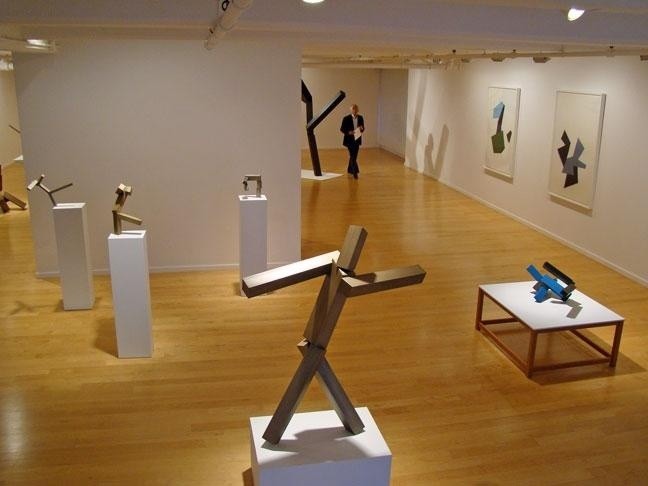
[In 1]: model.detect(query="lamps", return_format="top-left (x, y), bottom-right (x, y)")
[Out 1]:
top-left (201, 0), bottom-right (249, 51)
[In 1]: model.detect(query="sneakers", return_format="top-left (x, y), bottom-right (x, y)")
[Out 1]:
top-left (348, 169), bottom-right (358, 179)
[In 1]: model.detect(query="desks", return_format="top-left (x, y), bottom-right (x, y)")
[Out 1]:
top-left (474, 278), bottom-right (625, 378)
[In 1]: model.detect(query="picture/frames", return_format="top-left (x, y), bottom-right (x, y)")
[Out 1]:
top-left (482, 86), bottom-right (520, 179)
top-left (548, 88), bottom-right (607, 211)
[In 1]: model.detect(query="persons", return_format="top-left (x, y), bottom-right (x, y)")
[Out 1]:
top-left (339, 104), bottom-right (365, 179)
